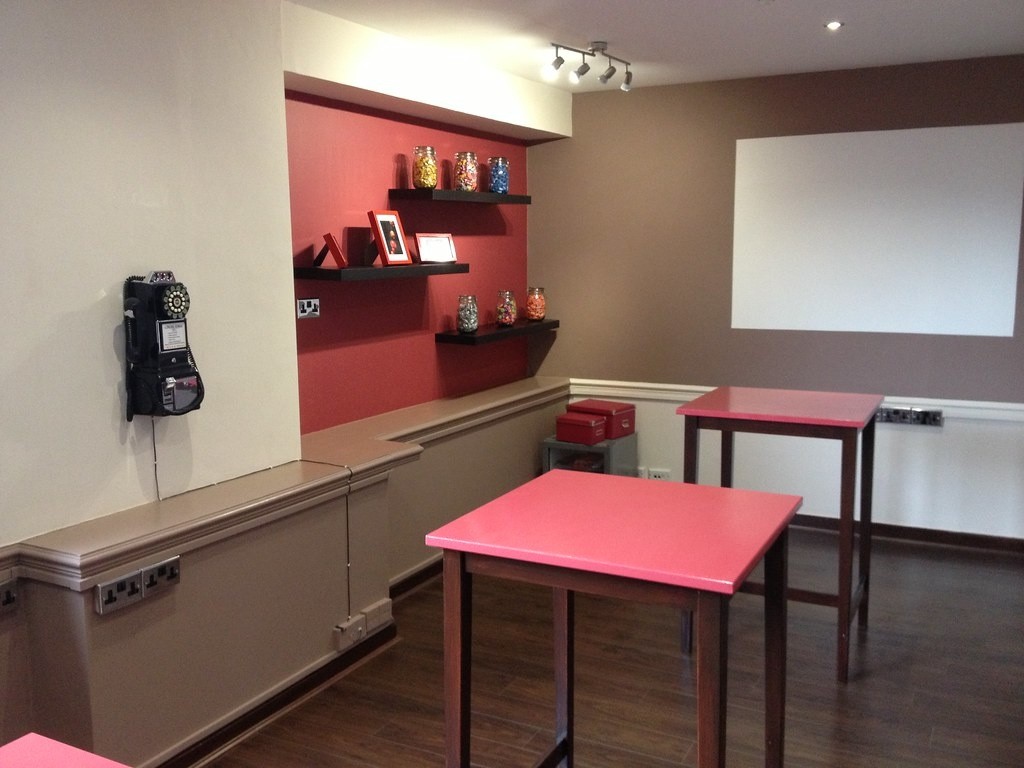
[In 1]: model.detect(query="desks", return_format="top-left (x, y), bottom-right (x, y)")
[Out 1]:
top-left (677, 386), bottom-right (885, 682)
top-left (426, 469), bottom-right (803, 768)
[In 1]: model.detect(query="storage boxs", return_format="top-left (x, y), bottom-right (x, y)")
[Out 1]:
top-left (565, 399), bottom-right (636, 439)
top-left (555, 414), bottom-right (606, 446)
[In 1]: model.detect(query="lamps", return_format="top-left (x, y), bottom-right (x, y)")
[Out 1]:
top-left (544, 41), bottom-right (632, 91)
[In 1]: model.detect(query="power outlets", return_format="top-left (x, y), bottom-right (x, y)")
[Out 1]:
top-left (649, 469), bottom-right (672, 482)
top-left (622, 467), bottom-right (648, 480)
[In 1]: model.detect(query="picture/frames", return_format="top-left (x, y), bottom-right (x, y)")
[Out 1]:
top-left (414, 233), bottom-right (458, 263)
top-left (314, 233), bottom-right (349, 268)
top-left (365, 210), bottom-right (413, 266)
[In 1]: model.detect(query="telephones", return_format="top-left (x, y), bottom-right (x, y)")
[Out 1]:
top-left (124, 270), bottom-right (206, 422)
